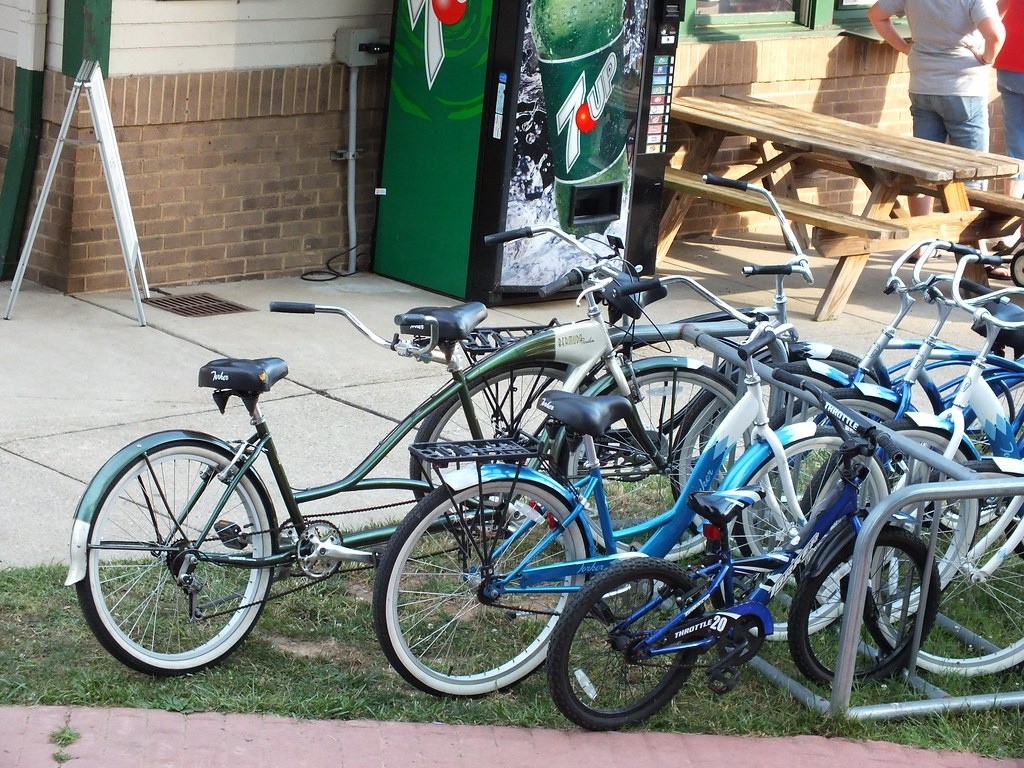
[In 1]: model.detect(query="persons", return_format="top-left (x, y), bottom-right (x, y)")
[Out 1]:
top-left (867, 0), bottom-right (1011, 282)
top-left (995, 0), bottom-right (1024, 160)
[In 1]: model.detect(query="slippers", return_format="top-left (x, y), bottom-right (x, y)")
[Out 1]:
top-left (984, 264), bottom-right (1014, 280)
top-left (908, 247), bottom-right (941, 265)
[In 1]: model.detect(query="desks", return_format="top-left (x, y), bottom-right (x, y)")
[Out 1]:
top-left (667, 89), bottom-right (1023, 324)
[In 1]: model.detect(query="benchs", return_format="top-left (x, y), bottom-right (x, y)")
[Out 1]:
top-left (748, 139), bottom-right (1024, 253)
top-left (655, 166), bottom-right (910, 324)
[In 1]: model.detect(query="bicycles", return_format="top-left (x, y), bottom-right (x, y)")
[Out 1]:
top-left (61, 173), bottom-right (1024, 731)
